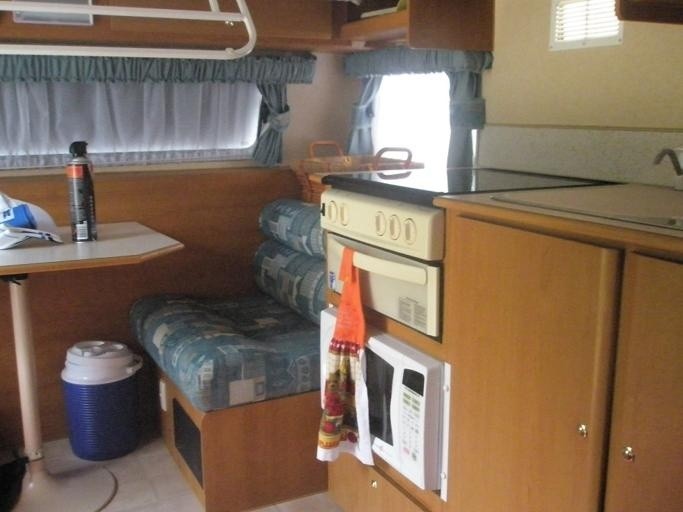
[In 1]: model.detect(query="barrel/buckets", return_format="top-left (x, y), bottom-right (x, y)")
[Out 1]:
top-left (61, 340), bottom-right (144, 461)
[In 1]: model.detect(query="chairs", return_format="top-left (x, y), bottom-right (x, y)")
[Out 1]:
top-left (125, 198), bottom-right (327, 511)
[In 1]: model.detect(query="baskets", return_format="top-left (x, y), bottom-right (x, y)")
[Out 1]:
top-left (290, 155), bottom-right (424, 205)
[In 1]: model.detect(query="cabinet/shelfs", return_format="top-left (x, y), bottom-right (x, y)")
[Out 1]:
top-left (0, 0), bottom-right (495, 53)
top-left (431, 186), bottom-right (683, 512)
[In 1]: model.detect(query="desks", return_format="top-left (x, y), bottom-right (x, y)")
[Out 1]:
top-left (0, 219), bottom-right (183, 512)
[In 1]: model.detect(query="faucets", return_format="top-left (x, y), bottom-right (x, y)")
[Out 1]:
top-left (653, 150), bottom-right (683, 176)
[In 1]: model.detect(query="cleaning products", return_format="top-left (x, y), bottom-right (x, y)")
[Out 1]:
top-left (66, 141), bottom-right (98, 242)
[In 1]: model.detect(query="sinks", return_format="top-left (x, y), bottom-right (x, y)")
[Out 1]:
top-left (490, 181), bottom-right (683, 235)
